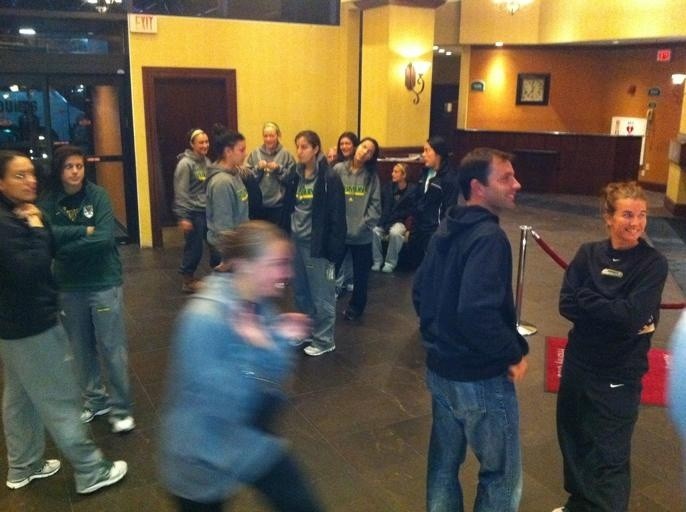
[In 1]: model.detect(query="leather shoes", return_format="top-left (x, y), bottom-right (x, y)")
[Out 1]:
top-left (343, 306), bottom-right (358, 321)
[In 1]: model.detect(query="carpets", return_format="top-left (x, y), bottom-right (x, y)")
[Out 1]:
top-left (544, 335), bottom-right (672, 407)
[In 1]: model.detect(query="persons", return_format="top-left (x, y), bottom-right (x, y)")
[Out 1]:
top-left (0, 148), bottom-right (129, 494)
top-left (149, 222), bottom-right (330, 512)
top-left (172, 128), bottom-right (212, 292)
top-left (34, 146), bottom-right (137, 435)
top-left (413, 135), bottom-right (460, 276)
top-left (281, 130), bottom-right (380, 358)
top-left (71, 115), bottom-right (97, 171)
top-left (550, 182), bottom-right (669, 512)
top-left (408, 145), bottom-right (529, 512)
top-left (371, 163), bottom-right (417, 271)
top-left (204, 121), bottom-right (296, 266)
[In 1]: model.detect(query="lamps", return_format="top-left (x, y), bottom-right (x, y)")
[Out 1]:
top-left (405, 61), bottom-right (429, 104)
top-left (490, 0), bottom-right (538, 15)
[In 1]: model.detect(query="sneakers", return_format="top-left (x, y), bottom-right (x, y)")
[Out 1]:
top-left (182, 272), bottom-right (195, 292)
top-left (302, 342), bottom-right (336, 357)
top-left (81, 403), bottom-right (112, 423)
top-left (287, 333), bottom-right (312, 347)
top-left (371, 261), bottom-right (383, 272)
top-left (76, 460), bottom-right (128, 494)
top-left (108, 414), bottom-right (135, 434)
top-left (381, 264), bottom-right (396, 273)
top-left (5, 458), bottom-right (61, 490)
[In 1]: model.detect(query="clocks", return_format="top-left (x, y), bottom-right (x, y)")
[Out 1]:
top-left (515, 73), bottom-right (551, 106)
